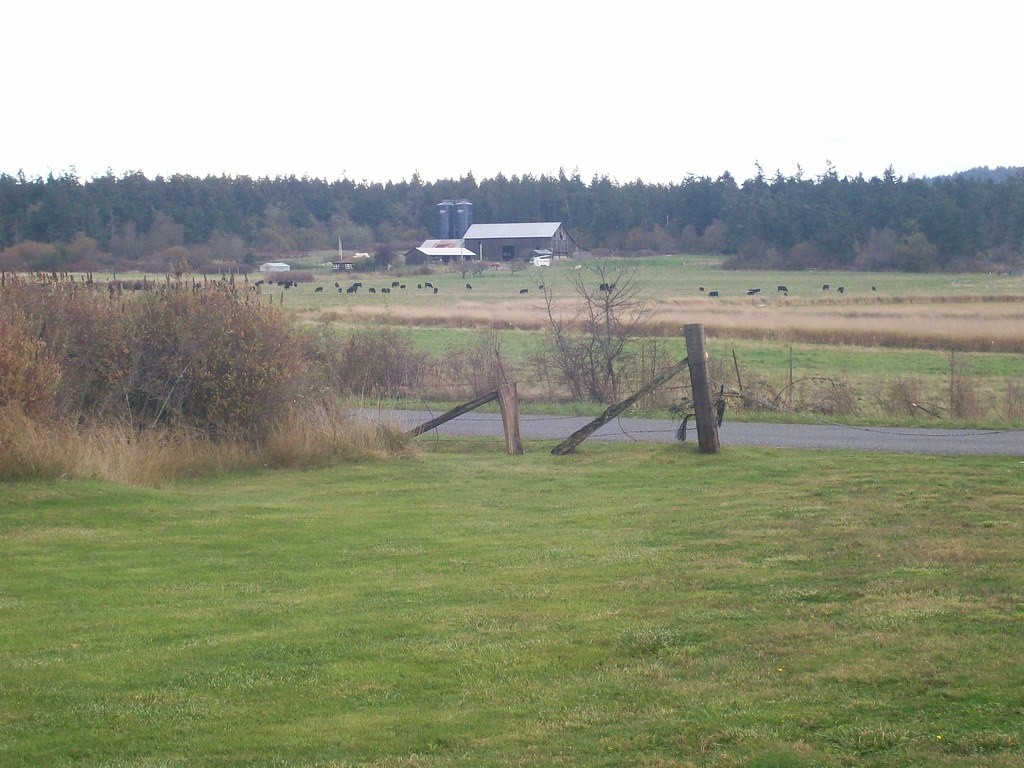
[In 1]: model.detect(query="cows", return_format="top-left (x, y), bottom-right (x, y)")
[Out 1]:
top-left (872, 286), bottom-right (876, 291)
top-left (699, 286), bottom-right (719, 298)
top-left (539, 284), bottom-right (544, 289)
top-left (519, 288), bottom-right (528, 294)
top-left (778, 285), bottom-right (788, 297)
top-left (465, 283), bottom-right (473, 289)
top-left (746, 288), bottom-right (761, 296)
top-left (315, 282), bottom-right (438, 294)
top-left (822, 283), bottom-right (845, 294)
top-left (599, 283), bottom-right (616, 291)
top-left (254, 280), bottom-right (297, 290)
